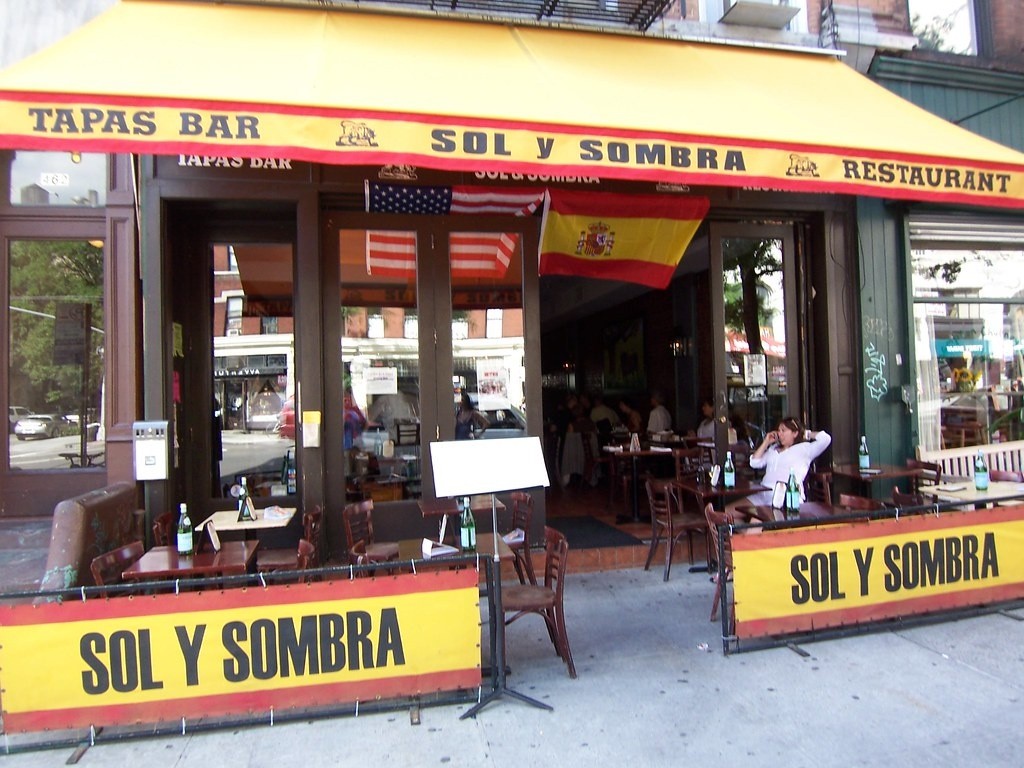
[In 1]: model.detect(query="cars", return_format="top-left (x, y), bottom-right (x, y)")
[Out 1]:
top-left (936, 384), bottom-right (1024, 438)
top-left (8, 406), bottom-right (98, 440)
top-left (275, 390), bottom-right (527, 440)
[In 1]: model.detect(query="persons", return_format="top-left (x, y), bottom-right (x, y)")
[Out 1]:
top-left (344, 387), bottom-right (368, 450)
top-left (709, 416), bottom-right (832, 583)
top-left (455, 393), bottom-right (490, 439)
top-left (540, 394), bottom-right (715, 441)
top-left (937, 358), bottom-right (956, 392)
top-left (362, 391), bottom-right (415, 443)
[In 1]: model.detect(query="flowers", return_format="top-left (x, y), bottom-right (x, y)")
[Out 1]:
top-left (951, 368), bottom-right (983, 392)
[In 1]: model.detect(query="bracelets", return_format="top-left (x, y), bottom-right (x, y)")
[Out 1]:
top-left (805, 430), bottom-right (811, 440)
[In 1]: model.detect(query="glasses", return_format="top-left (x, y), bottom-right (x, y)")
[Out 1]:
top-left (787, 417), bottom-right (799, 429)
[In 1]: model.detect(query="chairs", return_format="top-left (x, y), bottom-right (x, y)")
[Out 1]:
top-left (90, 421), bottom-right (1024, 677)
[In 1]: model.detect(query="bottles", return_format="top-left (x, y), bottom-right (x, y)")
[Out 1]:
top-left (374, 428), bottom-right (383, 457)
top-left (281, 455), bottom-right (288, 485)
top-left (786, 466), bottom-right (800, 512)
top-left (974, 448), bottom-right (988, 490)
top-left (460, 496), bottom-right (477, 551)
top-left (724, 451), bottom-right (735, 489)
top-left (406, 451), bottom-right (416, 481)
top-left (176, 503), bottom-right (194, 556)
top-left (238, 476), bottom-right (250, 517)
top-left (858, 435), bottom-right (870, 469)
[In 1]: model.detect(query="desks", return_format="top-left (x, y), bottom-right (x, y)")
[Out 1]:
top-left (395, 419), bottom-right (419, 446)
top-left (194, 507), bottom-right (297, 586)
top-left (417, 493), bottom-right (509, 534)
top-left (121, 538), bottom-right (259, 594)
top-left (603, 441), bottom-right (673, 523)
top-left (374, 471), bottom-right (420, 503)
top-left (397, 532), bottom-right (517, 567)
top-left (670, 435), bottom-right (713, 448)
top-left (833, 463), bottom-right (923, 497)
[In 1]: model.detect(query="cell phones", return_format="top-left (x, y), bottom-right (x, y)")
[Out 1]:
top-left (774, 434), bottom-right (779, 441)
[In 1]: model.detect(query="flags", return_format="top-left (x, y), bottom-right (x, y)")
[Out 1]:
top-left (537, 188), bottom-right (710, 290)
top-left (366, 231), bottom-right (519, 279)
top-left (364, 179), bottom-right (546, 217)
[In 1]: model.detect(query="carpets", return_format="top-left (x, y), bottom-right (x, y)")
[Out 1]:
top-left (544, 514), bottom-right (642, 549)
